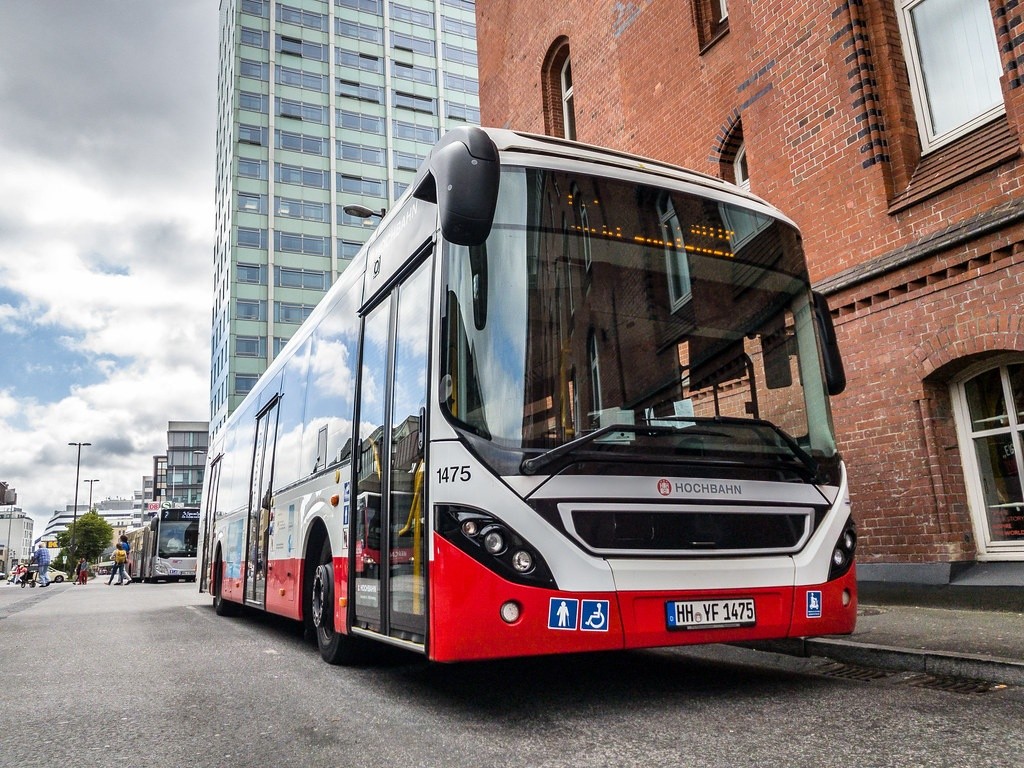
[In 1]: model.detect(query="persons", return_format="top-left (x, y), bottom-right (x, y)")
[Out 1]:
top-left (30, 544), bottom-right (50, 587)
top-left (11, 565), bottom-right (27, 583)
top-left (167, 533), bottom-right (183, 552)
top-left (114, 535), bottom-right (132, 585)
top-left (73, 557), bottom-right (88, 585)
top-left (104, 543), bottom-right (128, 585)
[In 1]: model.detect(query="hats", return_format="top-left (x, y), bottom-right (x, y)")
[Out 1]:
top-left (20, 565), bottom-right (24, 568)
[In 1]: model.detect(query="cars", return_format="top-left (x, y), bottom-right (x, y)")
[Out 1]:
top-left (7, 562), bottom-right (68, 583)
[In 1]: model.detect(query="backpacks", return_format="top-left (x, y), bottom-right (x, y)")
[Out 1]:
top-left (115, 549), bottom-right (126, 563)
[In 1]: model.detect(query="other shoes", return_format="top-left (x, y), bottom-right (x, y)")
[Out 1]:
top-left (114, 583), bottom-right (121, 585)
top-left (104, 582), bottom-right (110, 585)
top-left (39, 585), bottom-right (46, 587)
top-left (124, 580), bottom-right (133, 585)
top-left (73, 582), bottom-right (86, 585)
top-left (46, 582), bottom-right (50, 586)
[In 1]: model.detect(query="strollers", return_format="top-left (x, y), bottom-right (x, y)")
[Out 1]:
top-left (20, 563), bottom-right (39, 588)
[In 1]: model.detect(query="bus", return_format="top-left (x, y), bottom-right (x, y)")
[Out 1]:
top-left (194, 124), bottom-right (858, 664)
top-left (118, 508), bottom-right (201, 583)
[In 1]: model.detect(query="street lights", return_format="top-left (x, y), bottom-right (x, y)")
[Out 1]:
top-left (68, 442), bottom-right (91, 580)
top-left (84, 479), bottom-right (100, 561)
